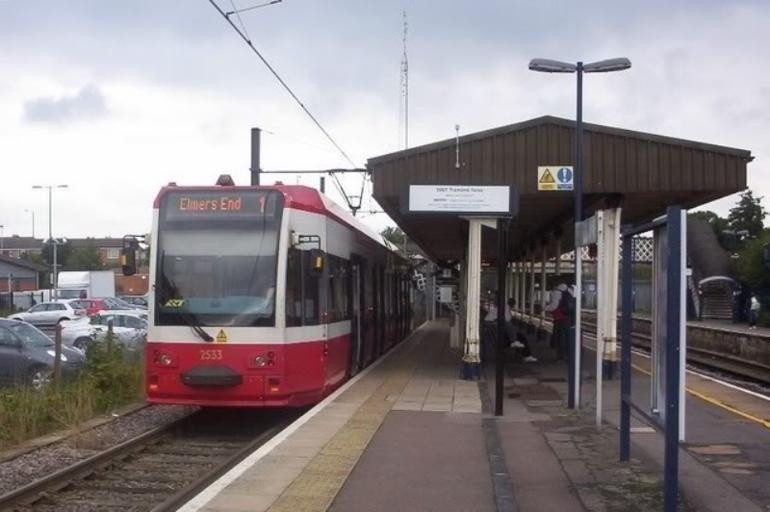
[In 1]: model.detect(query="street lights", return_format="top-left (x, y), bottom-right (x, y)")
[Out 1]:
top-left (31, 183), bottom-right (69, 286)
top-left (529, 57), bottom-right (633, 414)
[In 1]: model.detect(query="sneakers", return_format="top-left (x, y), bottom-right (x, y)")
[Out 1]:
top-left (510, 341), bottom-right (525, 348)
top-left (522, 356), bottom-right (537, 362)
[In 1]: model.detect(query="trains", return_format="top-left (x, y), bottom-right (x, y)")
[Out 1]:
top-left (120, 128), bottom-right (416, 410)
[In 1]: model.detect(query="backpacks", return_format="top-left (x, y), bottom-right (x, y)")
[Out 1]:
top-left (553, 287), bottom-right (575, 313)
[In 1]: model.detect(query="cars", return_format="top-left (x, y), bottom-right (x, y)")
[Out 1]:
top-left (0, 268), bottom-right (149, 395)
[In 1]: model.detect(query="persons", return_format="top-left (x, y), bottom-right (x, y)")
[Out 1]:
top-left (484, 298), bottom-right (515, 330)
top-left (544, 276), bottom-right (576, 363)
top-left (744, 289), bottom-right (762, 331)
top-left (504, 327), bottom-right (540, 363)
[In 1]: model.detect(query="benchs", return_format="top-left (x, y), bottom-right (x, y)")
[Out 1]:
top-left (489, 330), bottom-right (522, 364)
